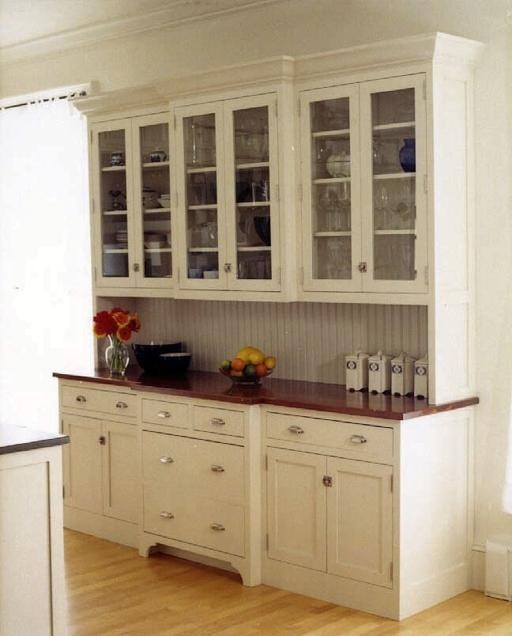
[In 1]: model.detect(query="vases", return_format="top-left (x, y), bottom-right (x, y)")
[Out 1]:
top-left (104, 335), bottom-right (130, 377)
top-left (399, 137), bottom-right (416, 173)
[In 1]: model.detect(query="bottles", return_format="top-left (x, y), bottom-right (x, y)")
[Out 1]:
top-left (184, 123), bottom-right (213, 165)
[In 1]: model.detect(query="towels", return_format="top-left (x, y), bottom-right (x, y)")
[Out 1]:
top-left (483, 540), bottom-right (511, 601)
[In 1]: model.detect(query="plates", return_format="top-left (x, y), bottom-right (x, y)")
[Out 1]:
top-left (115, 228), bottom-right (128, 249)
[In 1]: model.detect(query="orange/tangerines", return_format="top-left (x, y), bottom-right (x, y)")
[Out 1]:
top-left (221, 359), bottom-right (231, 371)
top-left (245, 364), bottom-right (255, 376)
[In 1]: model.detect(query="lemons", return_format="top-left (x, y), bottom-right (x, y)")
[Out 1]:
top-left (264, 356), bottom-right (276, 370)
top-left (249, 352), bottom-right (265, 364)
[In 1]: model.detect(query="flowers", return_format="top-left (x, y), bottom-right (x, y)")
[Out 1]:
top-left (92, 304), bottom-right (141, 342)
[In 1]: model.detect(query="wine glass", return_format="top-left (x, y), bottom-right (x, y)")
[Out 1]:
top-left (109, 183), bottom-right (122, 210)
top-left (316, 182), bottom-right (351, 232)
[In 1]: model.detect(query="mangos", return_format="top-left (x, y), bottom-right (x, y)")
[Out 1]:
top-left (237, 347), bottom-right (260, 363)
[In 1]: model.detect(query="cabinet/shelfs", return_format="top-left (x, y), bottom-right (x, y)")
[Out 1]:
top-left (69, 77), bottom-right (175, 300)
top-left (174, 55), bottom-right (290, 303)
top-left (259, 396), bottom-right (480, 623)
top-left (52, 372), bottom-right (141, 556)
top-left (132, 382), bottom-right (263, 588)
top-left (0, 426), bottom-right (72, 636)
top-left (294, 30), bottom-right (485, 306)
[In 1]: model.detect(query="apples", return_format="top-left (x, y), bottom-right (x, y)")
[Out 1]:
top-left (231, 358), bottom-right (245, 371)
top-left (256, 364), bottom-right (267, 376)
top-left (230, 370), bottom-right (243, 377)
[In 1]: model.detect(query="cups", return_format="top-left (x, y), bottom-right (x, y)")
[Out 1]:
top-left (112, 151), bottom-right (123, 165)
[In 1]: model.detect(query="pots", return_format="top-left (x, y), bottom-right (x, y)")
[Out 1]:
top-left (144, 186), bottom-right (158, 208)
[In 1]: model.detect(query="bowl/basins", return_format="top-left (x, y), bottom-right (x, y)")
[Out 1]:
top-left (203, 271), bottom-right (218, 279)
top-left (157, 194), bottom-right (170, 207)
top-left (189, 269), bottom-right (202, 277)
top-left (251, 216), bottom-right (270, 246)
top-left (143, 231), bottom-right (166, 249)
top-left (132, 341), bottom-right (180, 373)
top-left (161, 353), bottom-right (192, 371)
